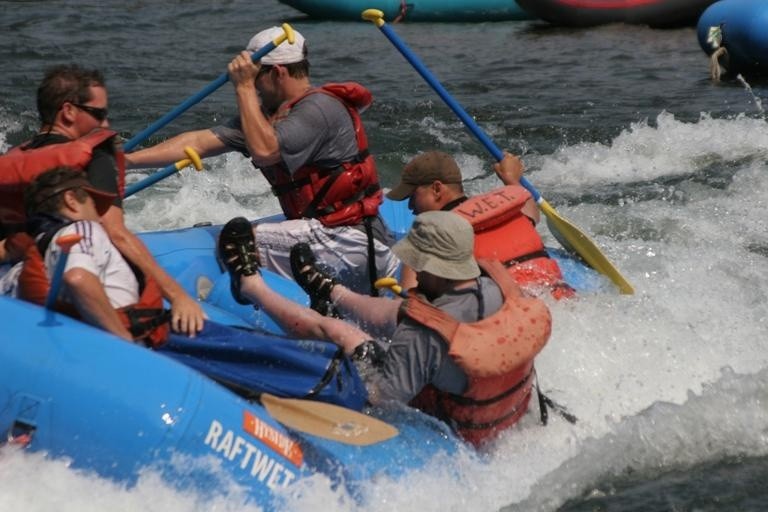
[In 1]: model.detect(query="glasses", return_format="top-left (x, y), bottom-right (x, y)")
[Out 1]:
top-left (77, 103), bottom-right (108, 122)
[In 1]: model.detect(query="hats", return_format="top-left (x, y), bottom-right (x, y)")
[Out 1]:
top-left (27, 165), bottom-right (118, 218)
top-left (386, 153), bottom-right (462, 202)
top-left (247, 25), bottom-right (306, 66)
top-left (391, 209), bottom-right (482, 281)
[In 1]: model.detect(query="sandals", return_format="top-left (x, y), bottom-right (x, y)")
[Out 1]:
top-left (290, 242), bottom-right (340, 319)
top-left (217, 216), bottom-right (262, 304)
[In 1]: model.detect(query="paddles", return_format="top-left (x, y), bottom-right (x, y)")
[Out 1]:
top-left (213, 369), bottom-right (400, 447)
top-left (362, 8), bottom-right (634, 297)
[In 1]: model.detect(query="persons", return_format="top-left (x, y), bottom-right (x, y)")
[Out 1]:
top-left (120, 21), bottom-right (405, 293)
top-left (22, 163), bottom-right (171, 346)
top-left (2, 57), bottom-right (211, 341)
top-left (214, 206), bottom-right (552, 450)
top-left (368, 142), bottom-right (583, 303)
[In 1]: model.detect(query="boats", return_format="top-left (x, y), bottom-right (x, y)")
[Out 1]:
top-left (514, 0), bottom-right (707, 24)
top-left (697, 0), bottom-right (768, 73)
top-left (0, 192), bottom-right (613, 511)
top-left (277, 0), bottom-right (528, 22)
